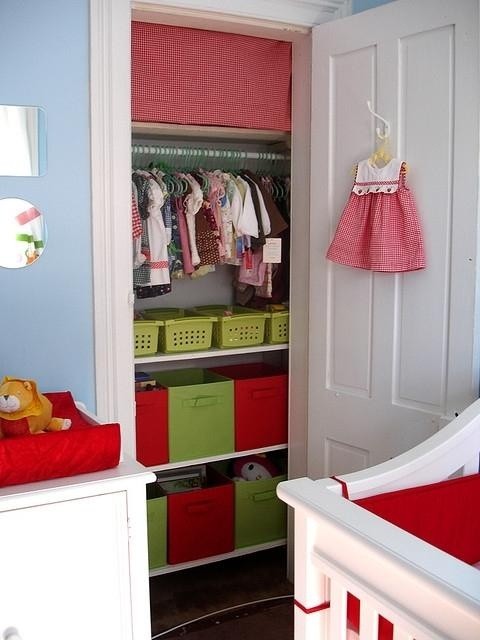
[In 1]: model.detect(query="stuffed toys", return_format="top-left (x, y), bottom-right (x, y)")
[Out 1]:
top-left (232, 452), bottom-right (281, 483)
top-left (0, 376), bottom-right (72, 434)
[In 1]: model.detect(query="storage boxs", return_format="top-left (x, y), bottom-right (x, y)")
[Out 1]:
top-left (160, 466), bottom-right (232, 566)
top-left (208, 358), bottom-right (289, 452)
top-left (152, 366), bottom-right (235, 464)
top-left (135, 383), bottom-right (169, 467)
top-left (228, 458), bottom-right (287, 549)
top-left (145, 482), bottom-right (168, 570)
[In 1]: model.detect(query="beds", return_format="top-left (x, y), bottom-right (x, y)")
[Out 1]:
top-left (276, 389), bottom-right (480, 640)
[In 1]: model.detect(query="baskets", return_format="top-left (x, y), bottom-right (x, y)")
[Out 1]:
top-left (128, 302), bottom-right (291, 354)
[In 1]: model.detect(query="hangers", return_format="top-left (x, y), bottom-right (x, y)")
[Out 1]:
top-left (353, 132), bottom-right (409, 178)
top-left (132, 142), bottom-right (293, 201)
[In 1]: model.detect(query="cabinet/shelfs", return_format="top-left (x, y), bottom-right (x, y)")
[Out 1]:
top-left (131, 22), bottom-right (289, 639)
top-left (1, 454), bottom-right (157, 640)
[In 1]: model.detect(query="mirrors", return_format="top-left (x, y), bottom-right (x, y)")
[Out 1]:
top-left (1, 106), bottom-right (47, 177)
top-left (0, 197), bottom-right (50, 271)
